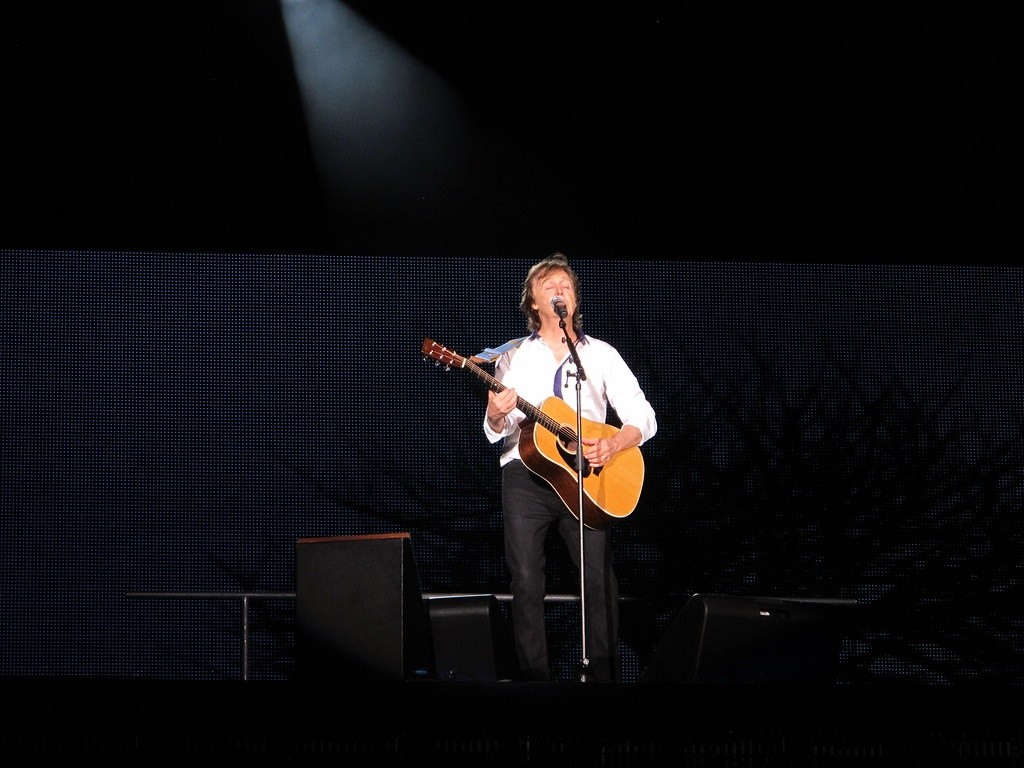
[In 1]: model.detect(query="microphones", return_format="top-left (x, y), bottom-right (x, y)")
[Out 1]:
top-left (551, 296), bottom-right (568, 318)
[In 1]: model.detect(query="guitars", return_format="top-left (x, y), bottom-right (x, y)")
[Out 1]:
top-left (419, 335), bottom-right (646, 534)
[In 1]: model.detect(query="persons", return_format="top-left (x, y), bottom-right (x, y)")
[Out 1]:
top-left (483, 253), bottom-right (658, 683)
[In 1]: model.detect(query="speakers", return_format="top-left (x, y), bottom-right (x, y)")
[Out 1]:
top-left (427, 596), bottom-right (524, 682)
top-left (633, 593), bottom-right (858, 690)
top-left (297, 539), bottom-right (436, 680)
